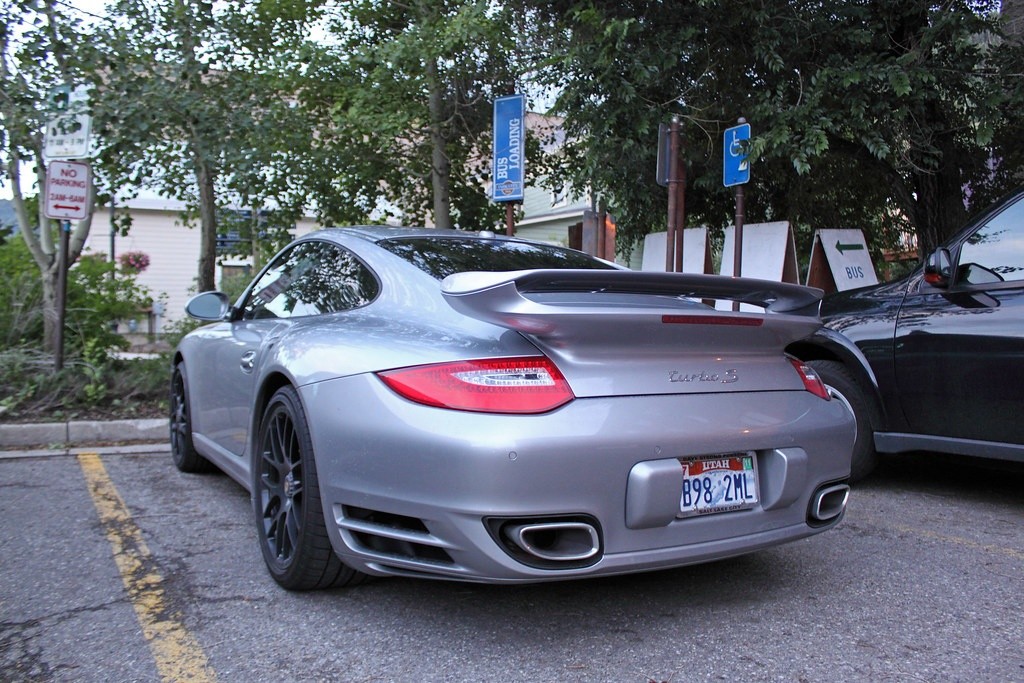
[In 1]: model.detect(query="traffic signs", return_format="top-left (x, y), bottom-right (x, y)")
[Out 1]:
top-left (44, 160), bottom-right (92, 221)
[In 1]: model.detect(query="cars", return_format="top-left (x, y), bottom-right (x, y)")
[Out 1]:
top-left (786, 183), bottom-right (1024, 485)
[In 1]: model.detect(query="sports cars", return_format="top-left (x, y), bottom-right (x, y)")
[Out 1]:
top-left (168, 223), bottom-right (860, 593)
